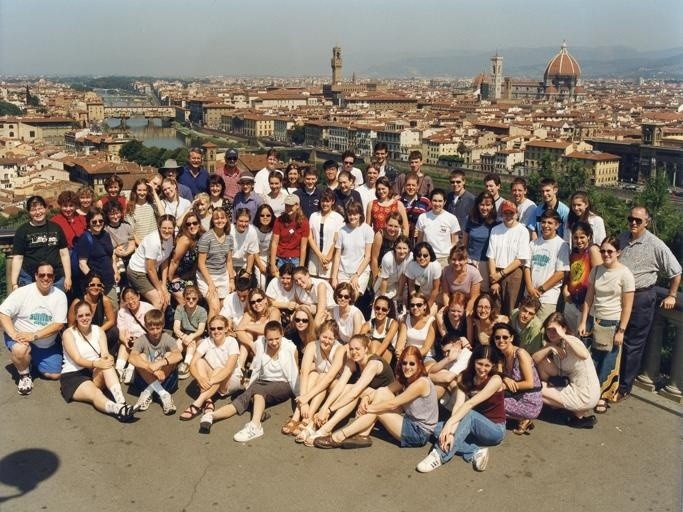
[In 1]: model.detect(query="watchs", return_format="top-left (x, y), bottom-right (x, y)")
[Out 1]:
top-left (618, 326), bottom-right (628, 333)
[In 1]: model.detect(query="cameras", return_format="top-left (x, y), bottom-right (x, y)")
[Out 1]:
top-left (129, 336), bottom-right (139, 348)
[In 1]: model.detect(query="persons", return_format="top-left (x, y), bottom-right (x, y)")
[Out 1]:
top-left (295, 333), bottom-right (397, 445)
top-left (1, 141), bottom-right (609, 392)
top-left (609, 203), bottom-right (682, 404)
top-left (489, 322), bottom-right (543, 435)
top-left (313, 345), bottom-right (439, 449)
top-left (578, 234), bottom-right (636, 414)
top-left (416, 344), bottom-right (506, 473)
top-left (128, 308), bottom-right (183, 415)
top-left (531, 311), bottom-right (599, 429)
top-left (179, 314), bottom-right (245, 421)
top-left (281, 318), bottom-right (348, 436)
top-left (60, 301), bottom-right (135, 422)
top-left (199, 320), bottom-right (300, 443)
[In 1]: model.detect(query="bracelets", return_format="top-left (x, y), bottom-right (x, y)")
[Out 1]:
top-left (666, 293), bottom-right (680, 300)
top-left (365, 394), bottom-right (372, 404)
top-left (447, 433), bottom-right (456, 437)
top-left (329, 408), bottom-right (334, 414)
